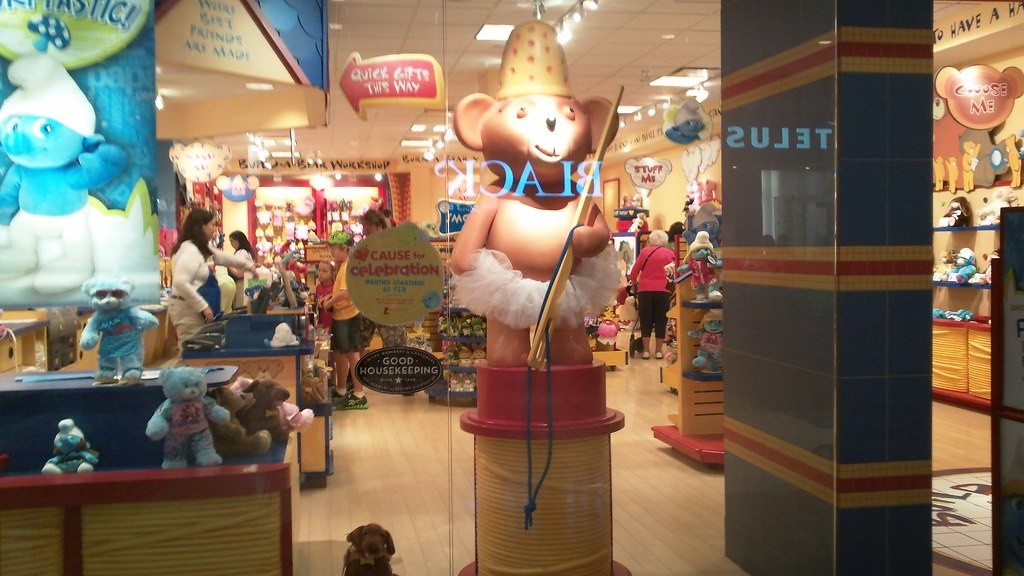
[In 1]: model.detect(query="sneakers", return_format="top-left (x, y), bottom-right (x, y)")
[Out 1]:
top-left (332, 393), bottom-right (347, 406)
top-left (336, 394), bottom-right (368, 410)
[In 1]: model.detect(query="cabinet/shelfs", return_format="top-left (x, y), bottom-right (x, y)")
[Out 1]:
top-left (176, 180), bottom-right (223, 248)
top-left (424, 308), bottom-right (487, 402)
top-left (651, 234), bottom-right (725, 469)
top-left (933, 226), bottom-right (1000, 410)
top-left (179, 309), bottom-right (335, 478)
top-left (0, 304), bottom-right (177, 372)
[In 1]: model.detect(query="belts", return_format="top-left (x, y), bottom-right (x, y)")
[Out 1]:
top-left (172, 295), bottom-right (184, 301)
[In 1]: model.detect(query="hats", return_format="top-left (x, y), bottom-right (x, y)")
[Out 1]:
top-left (322, 231), bottom-right (354, 246)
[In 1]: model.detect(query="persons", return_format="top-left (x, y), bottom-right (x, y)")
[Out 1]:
top-left (169, 206), bottom-right (260, 356)
top-left (317, 230), bottom-right (369, 410)
top-left (362, 210), bottom-right (416, 396)
top-left (315, 258), bottom-right (355, 395)
top-left (630, 229), bottom-right (675, 359)
top-left (228, 231), bottom-right (257, 310)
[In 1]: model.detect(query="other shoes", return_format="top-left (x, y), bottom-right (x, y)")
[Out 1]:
top-left (642, 351), bottom-right (650, 359)
top-left (655, 352), bottom-right (663, 359)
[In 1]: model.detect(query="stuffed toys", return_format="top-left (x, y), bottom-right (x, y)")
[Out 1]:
top-left (41, 420), bottom-right (98, 474)
top-left (932, 184), bottom-right (1017, 325)
top-left (253, 196), bottom-right (384, 307)
top-left (342, 523), bottom-right (400, 576)
top-left (673, 200), bottom-right (724, 373)
top-left (414, 263), bottom-right (487, 391)
top-left (145, 366), bottom-right (231, 469)
top-left (208, 370), bottom-right (313, 459)
top-left (81, 275), bottom-right (158, 383)
